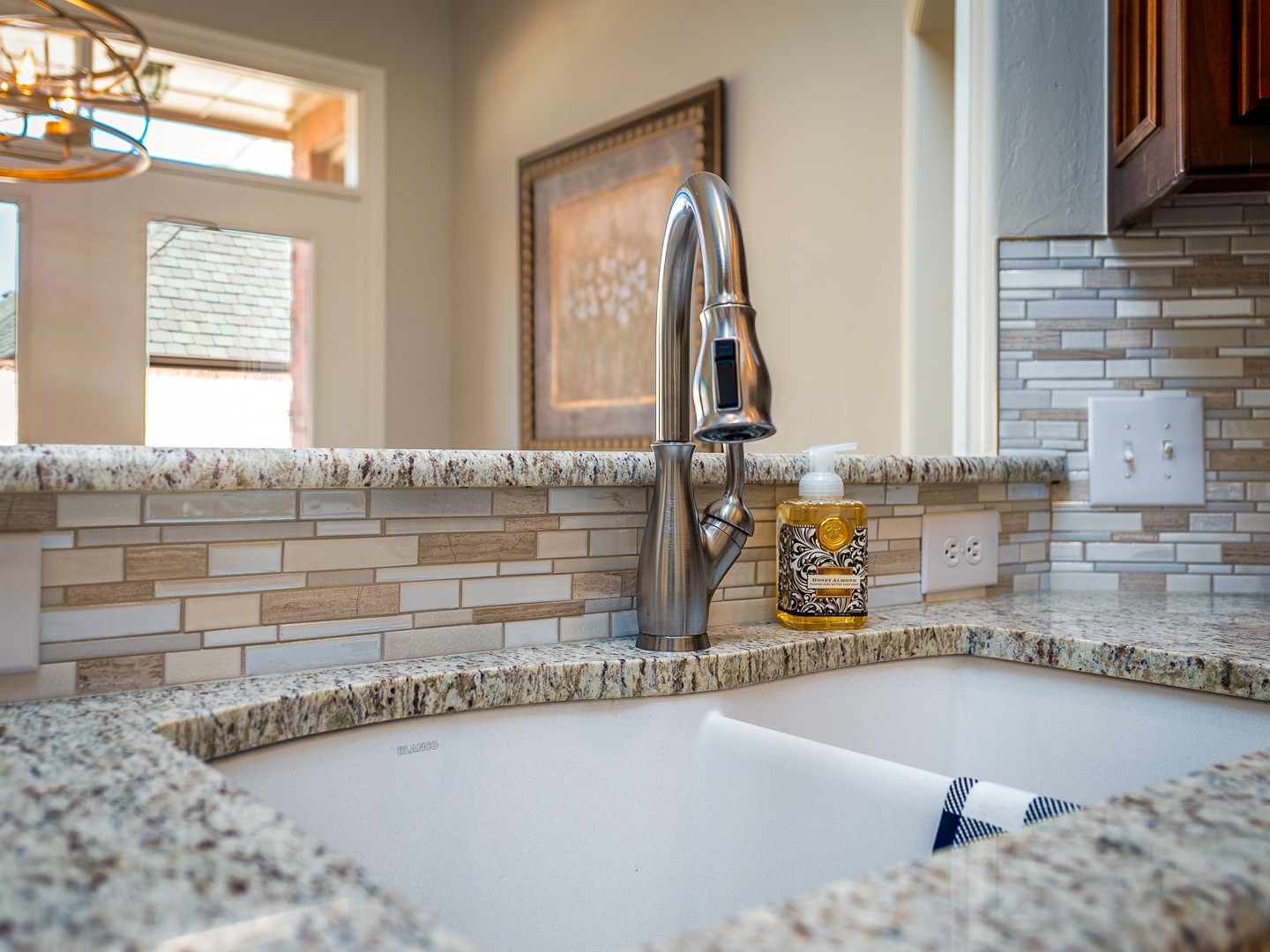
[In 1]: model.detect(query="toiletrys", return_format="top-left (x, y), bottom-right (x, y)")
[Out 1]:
top-left (771, 442), bottom-right (872, 632)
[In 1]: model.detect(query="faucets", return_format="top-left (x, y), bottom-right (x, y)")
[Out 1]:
top-left (632, 169), bottom-right (779, 655)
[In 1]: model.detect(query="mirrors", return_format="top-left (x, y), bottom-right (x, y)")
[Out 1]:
top-left (0, 0), bottom-right (999, 458)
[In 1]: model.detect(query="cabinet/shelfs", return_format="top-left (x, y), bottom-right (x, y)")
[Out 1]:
top-left (1110, 0), bottom-right (1270, 231)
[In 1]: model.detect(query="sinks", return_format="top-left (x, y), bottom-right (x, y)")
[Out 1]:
top-left (145, 658), bottom-right (1097, 952)
top-left (689, 615), bottom-right (1270, 848)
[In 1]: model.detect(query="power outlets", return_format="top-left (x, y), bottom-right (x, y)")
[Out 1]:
top-left (922, 510), bottom-right (999, 594)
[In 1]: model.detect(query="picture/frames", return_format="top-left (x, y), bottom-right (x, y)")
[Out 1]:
top-left (516, 79), bottom-right (724, 459)
